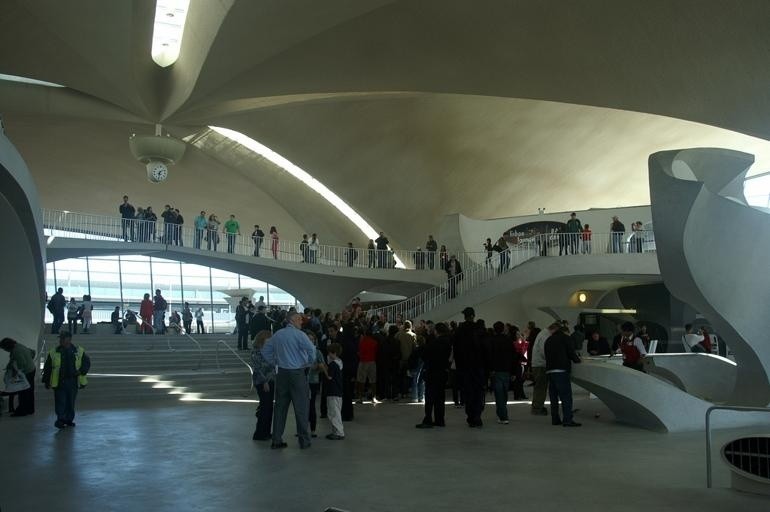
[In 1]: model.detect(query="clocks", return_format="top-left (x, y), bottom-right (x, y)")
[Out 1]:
top-left (144, 162), bottom-right (168, 183)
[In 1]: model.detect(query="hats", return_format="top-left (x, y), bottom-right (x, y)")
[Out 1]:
top-left (461, 307), bottom-right (475, 314)
top-left (57, 330), bottom-right (72, 337)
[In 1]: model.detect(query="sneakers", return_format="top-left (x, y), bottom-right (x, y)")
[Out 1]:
top-left (498, 420), bottom-right (509, 424)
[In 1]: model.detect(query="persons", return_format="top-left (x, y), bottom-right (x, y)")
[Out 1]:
top-left (194, 211), bottom-right (206, 249)
top-left (440, 245), bottom-right (447, 270)
top-left (507, 318), bottom-right (584, 427)
top-left (497, 232), bottom-right (511, 276)
top-left (538, 235), bottom-right (549, 256)
top-left (0, 337), bottom-right (36, 417)
top-left (43, 330), bottom-right (90, 429)
top-left (195, 307), bottom-right (205, 334)
top-left (631, 221), bottom-right (645, 253)
top-left (235, 295), bottom-right (364, 440)
top-left (556, 212), bottom-right (592, 256)
top-left (368, 232), bottom-right (390, 268)
top-left (416, 306), bottom-right (516, 430)
top-left (356, 304), bottom-right (426, 404)
top-left (485, 238), bottom-right (493, 270)
top-left (344, 242), bottom-right (356, 267)
top-left (300, 234), bottom-right (320, 264)
top-left (612, 216), bottom-right (625, 253)
top-left (445, 255), bottom-right (464, 299)
top-left (205, 214), bottom-right (221, 251)
top-left (587, 321), bottom-right (652, 372)
top-left (119, 196), bottom-right (135, 242)
top-left (260, 311), bottom-right (317, 449)
top-left (413, 246), bottom-right (425, 270)
top-left (140, 288), bottom-right (193, 334)
top-left (51, 287), bottom-right (66, 334)
top-left (426, 235), bottom-right (437, 269)
top-left (160, 204), bottom-right (183, 247)
top-left (681, 322), bottom-right (712, 353)
top-left (67, 295), bottom-right (93, 334)
top-left (270, 227), bottom-right (279, 259)
top-left (111, 306), bottom-right (127, 334)
top-left (251, 225), bottom-right (265, 256)
top-left (134, 207), bottom-right (158, 242)
top-left (223, 214), bottom-right (240, 253)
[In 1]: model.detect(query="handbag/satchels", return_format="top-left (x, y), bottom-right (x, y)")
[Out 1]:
top-left (5, 363), bottom-right (31, 393)
top-left (691, 344), bottom-right (706, 352)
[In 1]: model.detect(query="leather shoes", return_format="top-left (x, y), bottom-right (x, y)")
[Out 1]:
top-left (563, 422), bottom-right (581, 426)
top-left (328, 435), bottom-right (344, 440)
top-left (55, 420), bottom-right (65, 428)
top-left (272, 442), bottom-right (287, 449)
top-left (326, 434), bottom-right (334, 438)
top-left (416, 423), bottom-right (432, 428)
top-left (64, 420), bottom-right (75, 426)
